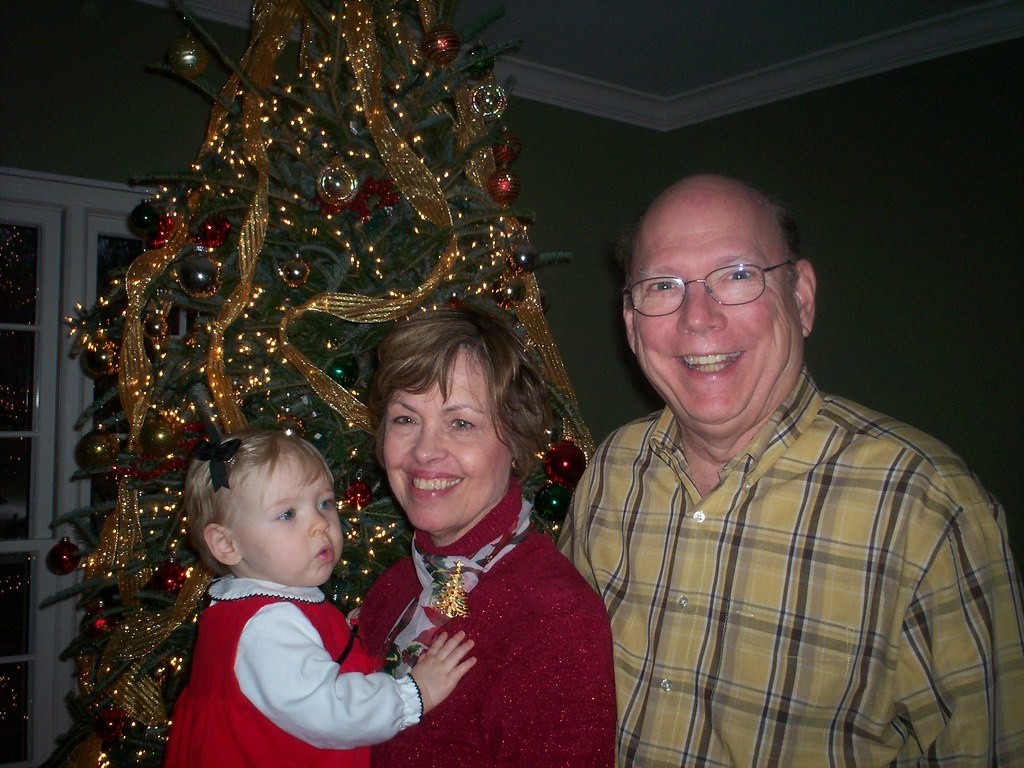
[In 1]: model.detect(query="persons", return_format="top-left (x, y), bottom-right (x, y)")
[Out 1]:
top-left (556, 174), bottom-right (1024, 768)
top-left (165, 432), bottom-right (478, 768)
top-left (355, 305), bottom-right (616, 768)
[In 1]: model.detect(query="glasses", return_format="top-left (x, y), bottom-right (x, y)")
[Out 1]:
top-left (622, 261), bottom-right (792, 317)
